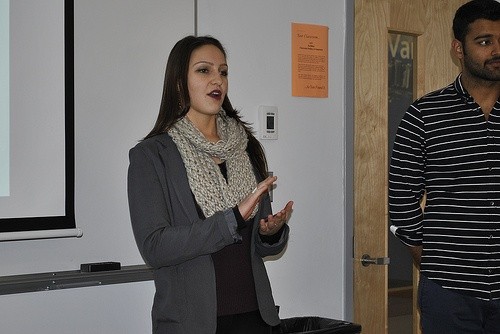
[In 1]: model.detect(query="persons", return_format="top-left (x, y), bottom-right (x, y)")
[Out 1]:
top-left (127, 35), bottom-right (294, 334)
top-left (388, 0), bottom-right (500, 334)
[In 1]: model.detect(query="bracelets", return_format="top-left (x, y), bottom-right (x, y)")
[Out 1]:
top-left (251, 185), bottom-right (257, 194)
